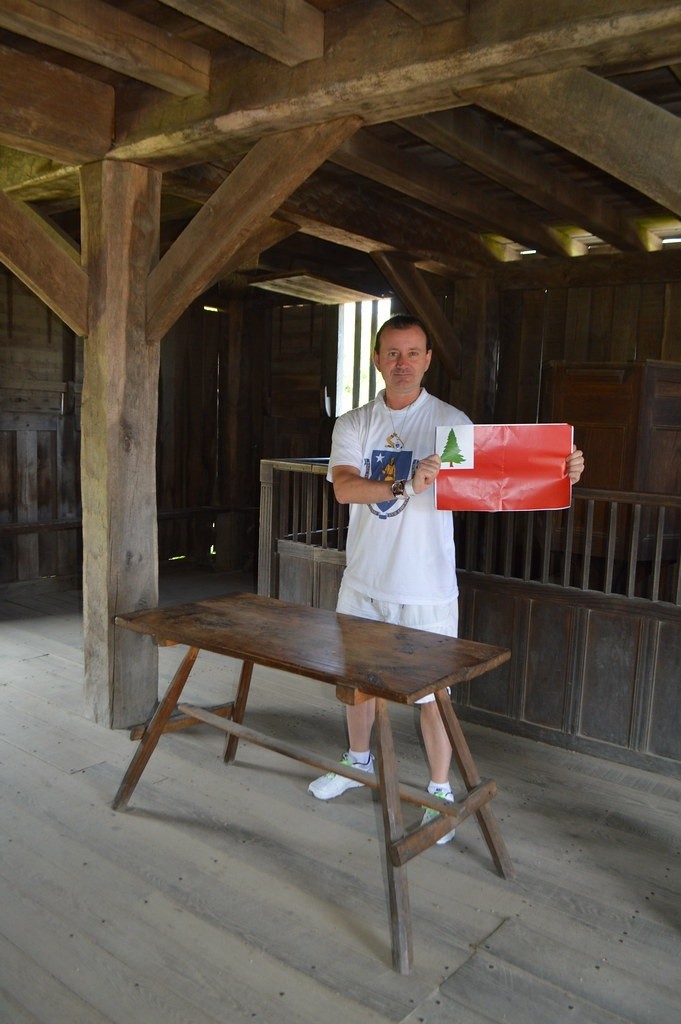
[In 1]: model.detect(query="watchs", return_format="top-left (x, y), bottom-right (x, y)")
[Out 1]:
top-left (392, 479), bottom-right (406, 500)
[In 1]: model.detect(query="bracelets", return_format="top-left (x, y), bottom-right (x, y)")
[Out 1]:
top-left (405, 479), bottom-right (416, 496)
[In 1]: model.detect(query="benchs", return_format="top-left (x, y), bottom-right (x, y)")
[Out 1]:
top-left (109, 594), bottom-right (511, 976)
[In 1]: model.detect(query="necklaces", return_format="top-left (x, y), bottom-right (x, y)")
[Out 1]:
top-left (389, 404), bottom-right (411, 449)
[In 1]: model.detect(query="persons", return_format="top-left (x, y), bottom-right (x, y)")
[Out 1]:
top-left (382, 457), bottom-right (395, 480)
top-left (306, 315), bottom-right (585, 844)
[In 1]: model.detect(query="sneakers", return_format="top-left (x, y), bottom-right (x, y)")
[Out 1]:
top-left (308, 752), bottom-right (375, 800)
top-left (421, 786), bottom-right (456, 843)
